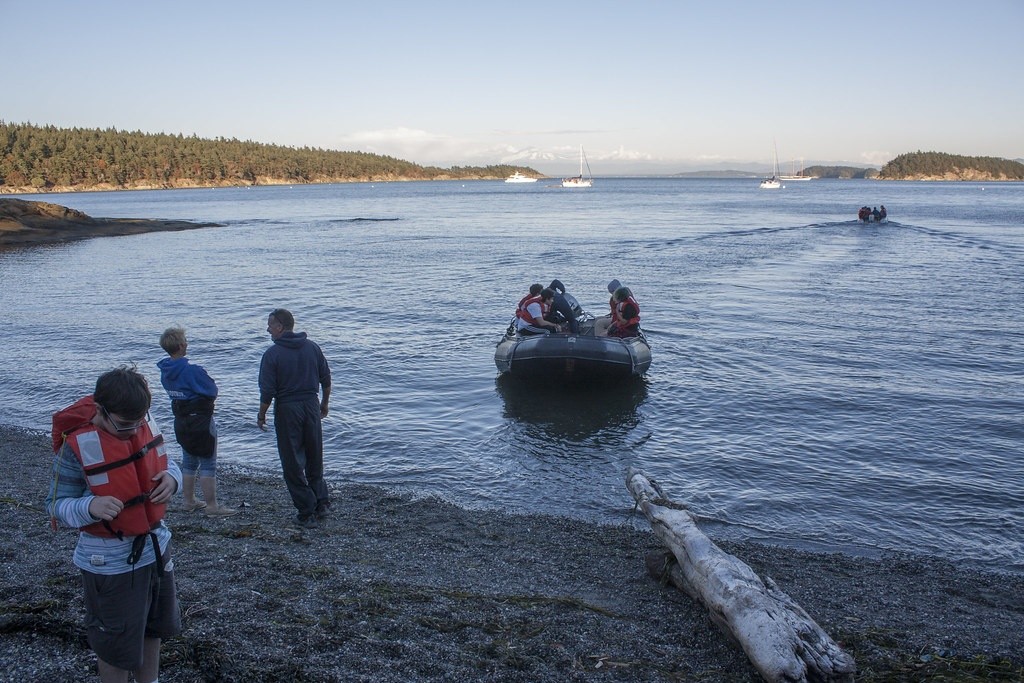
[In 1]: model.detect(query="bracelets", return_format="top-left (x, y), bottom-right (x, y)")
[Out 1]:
top-left (555, 325), bottom-right (560, 329)
top-left (257, 412), bottom-right (265, 420)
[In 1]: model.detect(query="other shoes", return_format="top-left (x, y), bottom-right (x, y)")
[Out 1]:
top-left (180, 499), bottom-right (207, 512)
top-left (317, 504), bottom-right (331, 516)
top-left (203, 505), bottom-right (240, 518)
top-left (293, 511), bottom-right (318, 528)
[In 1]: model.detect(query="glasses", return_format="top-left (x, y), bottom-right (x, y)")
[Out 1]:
top-left (101, 407), bottom-right (152, 432)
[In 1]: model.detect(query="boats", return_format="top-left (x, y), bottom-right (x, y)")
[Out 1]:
top-left (504, 171), bottom-right (538, 183)
top-left (780, 157), bottom-right (812, 181)
top-left (493, 308), bottom-right (652, 377)
top-left (857, 216), bottom-right (889, 224)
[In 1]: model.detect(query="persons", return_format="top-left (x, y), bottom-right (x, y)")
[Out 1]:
top-left (880, 205), bottom-right (887, 220)
top-left (608, 285), bottom-right (640, 338)
top-left (516, 284), bottom-right (544, 329)
top-left (517, 289), bottom-right (562, 335)
top-left (47, 364), bottom-right (183, 683)
top-left (155, 328), bottom-right (241, 517)
top-left (872, 207), bottom-right (880, 223)
top-left (859, 206), bottom-right (871, 223)
top-left (257, 309), bottom-right (331, 526)
top-left (595, 280), bottom-right (624, 337)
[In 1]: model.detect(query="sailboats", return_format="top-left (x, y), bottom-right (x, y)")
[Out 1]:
top-left (561, 144), bottom-right (594, 187)
top-left (759, 137), bottom-right (782, 189)
